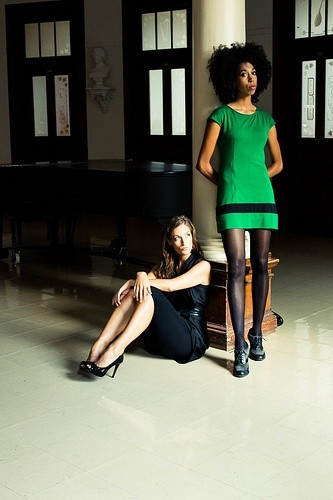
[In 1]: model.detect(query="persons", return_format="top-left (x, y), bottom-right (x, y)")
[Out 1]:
top-left (191, 41), bottom-right (287, 380)
top-left (76, 215), bottom-right (213, 380)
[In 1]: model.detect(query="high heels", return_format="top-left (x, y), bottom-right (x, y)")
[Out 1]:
top-left (79, 354), bottom-right (124, 378)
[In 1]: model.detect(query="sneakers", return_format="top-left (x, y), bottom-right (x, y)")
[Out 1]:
top-left (234, 342), bottom-right (249, 377)
top-left (248, 328), bottom-right (266, 361)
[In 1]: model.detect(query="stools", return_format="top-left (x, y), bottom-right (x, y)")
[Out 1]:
top-left (11, 213), bottom-right (61, 245)
